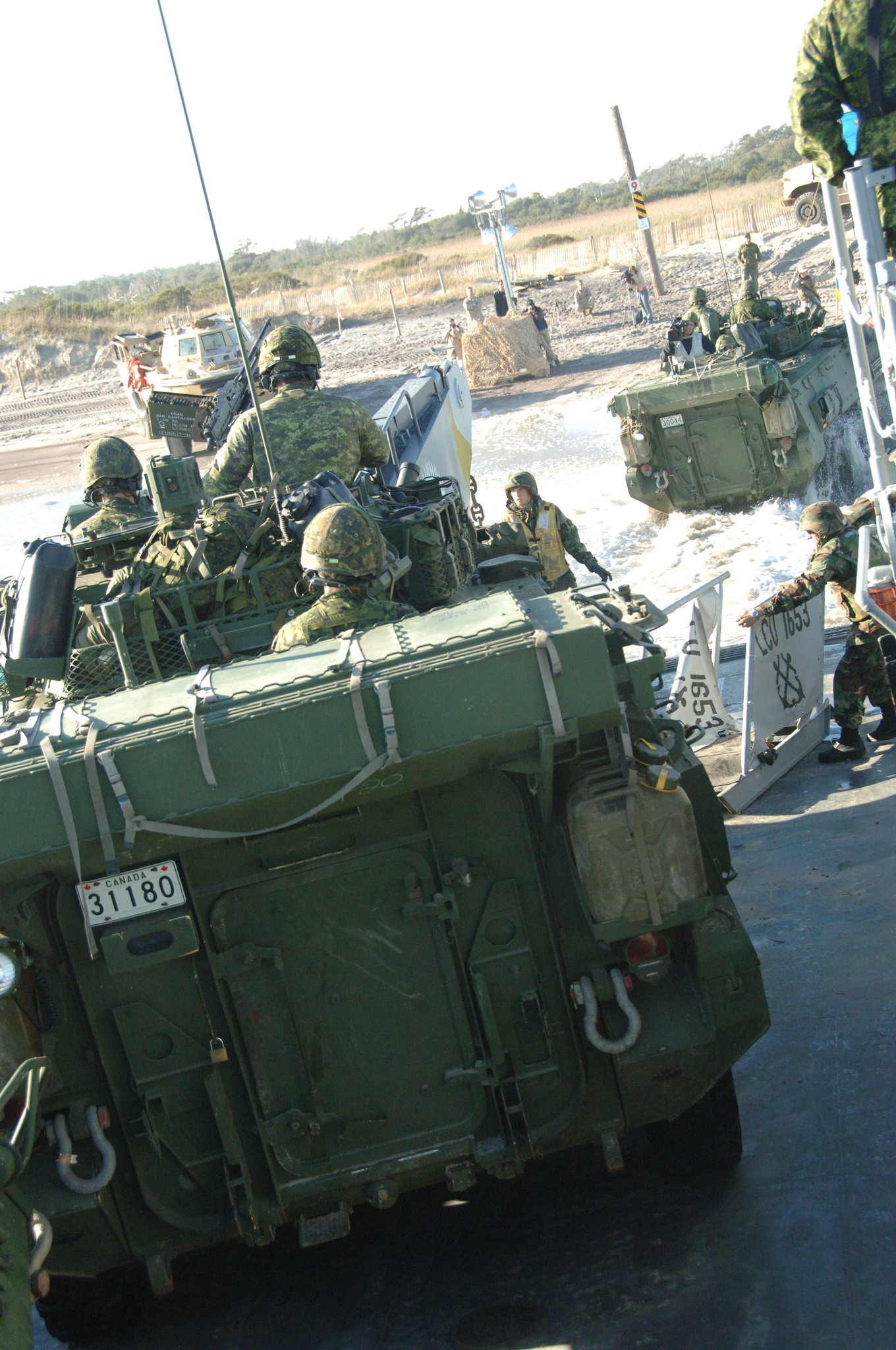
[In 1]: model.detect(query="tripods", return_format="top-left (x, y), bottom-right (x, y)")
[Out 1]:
top-left (622, 288), bottom-right (650, 328)
top-left (550, 301), bottom-right (580, 353)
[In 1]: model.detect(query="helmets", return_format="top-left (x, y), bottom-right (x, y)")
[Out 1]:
top-left (301, 502), bottom-right (386, 589)
top-left (78, 436), bottom-right (143, 495)
top-left (681, 311), bottom-right (698, 327)
top-left (715, 335), bottom-right (736, 351)
top-left (798, 501), bottom-right (847, 535)
top-left (689, 287), bottom-right (707, 305)
top-left (739, 280), bottom-right (759, 296)
top-left (505, 471), bottom-right (539, 500)
top-left (256, 325), bottom-right (321, 380)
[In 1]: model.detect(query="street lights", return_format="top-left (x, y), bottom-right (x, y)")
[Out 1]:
top-left (467, 182), bottom-right (518, 310)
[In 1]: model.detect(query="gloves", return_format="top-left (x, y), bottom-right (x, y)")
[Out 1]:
top-left (593, 565), bottom-right (612, 582)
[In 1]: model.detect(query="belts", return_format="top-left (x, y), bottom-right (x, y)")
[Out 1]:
top-left (856, 99), bottom-right (896, 125)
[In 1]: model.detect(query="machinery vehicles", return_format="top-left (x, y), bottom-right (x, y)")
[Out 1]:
top-left (0, 346), bottom-right (775, 1350)
top-left (111, 306), bottom-right (275, 458)
top-left (779, 153), bottom-right (850, 227)
top-left (606, 294), bottom-right (884, 513)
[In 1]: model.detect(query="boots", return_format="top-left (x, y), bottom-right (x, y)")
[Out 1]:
top-left (867, 700), bottom-right (896, 742)
top-left (818, 728), bottom-right (867, 763)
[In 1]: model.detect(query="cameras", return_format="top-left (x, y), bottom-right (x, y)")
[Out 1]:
top-left (530, 305), bottom-right (534, 310)
top-left (622, 270), bottom-right (634, 283)
top-left (665, 313), bottom-right (689, 341)
top-left (449, 323), bottom-right (456, 328)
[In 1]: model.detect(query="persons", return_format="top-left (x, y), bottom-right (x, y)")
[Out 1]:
top-left (727, 279), bottom-right (779, 326)
top-left (660, 311), bottom-right (717, 369)
top-left (443, 318), bottom-right (465, 361)
top-left (624, 266), bottom-right (653, 325)
top-left (520, 299), bottom-right (559, 368)
top-left (501, 471), bottom-right (612, 600)
top-left (788, 265), bottom-right (825, 334)
top-left (682, 287), bottom-right (726, 352)
top-left (203, 324), bottom-right (390, 521)
top-left (65, 436), bottom-right (159, 542)
top-left (493, 280), bottom-right (508, 318)
top-left (736, 501), bottom-right (896, 763)
top-left (271, 502), bottom-right (420, 653)
top-left (735, 232), bottom-right (761, 283)
top-left (788, 0), bottom-right (896, 266)
top-left (463, 287), bottom-right (485, 326)
top-left (574, 280), bottom-right (595, 318)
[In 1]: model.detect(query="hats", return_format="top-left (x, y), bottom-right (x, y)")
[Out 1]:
top-left (797, 264), bottom-right (808, 273)
top-left (744, 233), bottom-right (750, 238)
